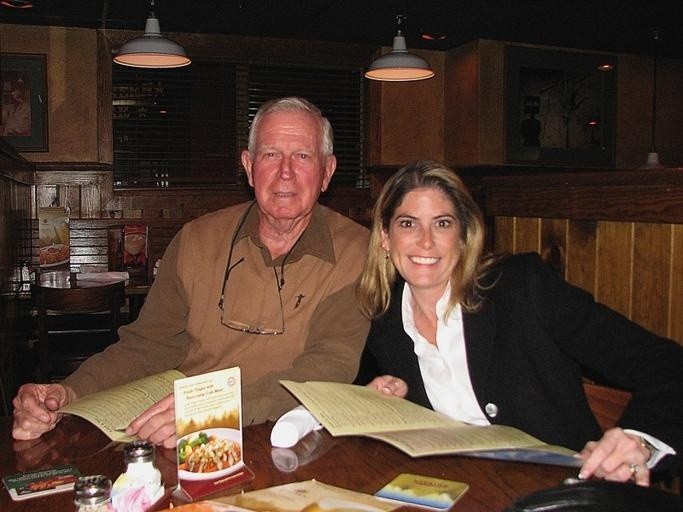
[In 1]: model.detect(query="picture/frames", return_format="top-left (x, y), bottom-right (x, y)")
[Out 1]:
top-left (500, 44), bottom-right (620, 169)
top-left (1, 53), bottom-right (51, 153)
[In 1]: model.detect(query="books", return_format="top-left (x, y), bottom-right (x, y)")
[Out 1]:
top-left (53, 368), bottom-right (188, 445)
top-left (276, 378), bottom-right (548, 459)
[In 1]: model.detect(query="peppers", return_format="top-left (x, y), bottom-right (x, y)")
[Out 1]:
top-left (178, 432), bottom-right (208, 464)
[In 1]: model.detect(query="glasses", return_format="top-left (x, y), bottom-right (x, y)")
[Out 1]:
top-left (219, 257), bottom-right (285, 335)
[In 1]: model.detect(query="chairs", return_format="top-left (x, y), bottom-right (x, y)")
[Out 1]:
top-left (29, 281), bottom-right (128, 387)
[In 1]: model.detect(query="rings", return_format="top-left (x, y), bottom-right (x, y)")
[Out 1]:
top-left (626, 463), bottom-right (643, 475)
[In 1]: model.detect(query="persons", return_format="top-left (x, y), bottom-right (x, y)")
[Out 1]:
top-left (10, 96), bottom-right (381, 451)
top-left (350, 155), bottom-right (683, 490)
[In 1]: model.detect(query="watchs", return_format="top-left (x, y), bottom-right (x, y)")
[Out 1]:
top-left (638, 435), bottom-right (656, 463)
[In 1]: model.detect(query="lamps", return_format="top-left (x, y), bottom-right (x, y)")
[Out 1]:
top-left (112, 2), bottom-right (194, 70)
top-left (363, 1), bottom-right (435, 83)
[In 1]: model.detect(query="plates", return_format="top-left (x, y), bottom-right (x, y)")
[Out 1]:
top-left (40, 243), bottom-right (70, 268)
top-left (176, 427), bottom-right (243, 480)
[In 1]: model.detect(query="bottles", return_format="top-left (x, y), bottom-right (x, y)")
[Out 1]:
top-left (74, 440), bottom-right (166, 511)
